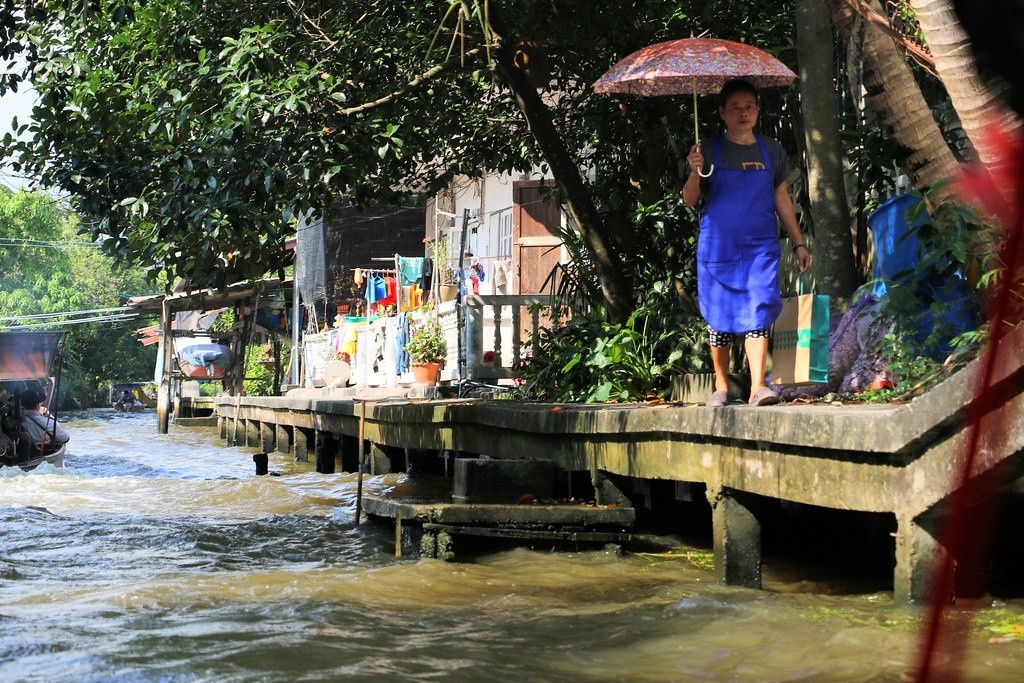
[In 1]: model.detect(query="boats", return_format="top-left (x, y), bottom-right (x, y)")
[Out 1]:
top-left (115, 402), bottom-right (148, 413)
top-left (0, 328), bottom-right (71, 472)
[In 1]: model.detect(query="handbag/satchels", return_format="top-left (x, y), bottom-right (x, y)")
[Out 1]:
top-left (771, 269), bottom-right (831, 386)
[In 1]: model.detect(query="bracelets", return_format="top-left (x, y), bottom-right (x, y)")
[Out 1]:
top-left (792, 244), bottom-right (807, 253)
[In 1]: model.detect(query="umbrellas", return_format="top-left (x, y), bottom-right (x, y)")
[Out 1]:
top-left (594, 38), bottom-right (799, 178)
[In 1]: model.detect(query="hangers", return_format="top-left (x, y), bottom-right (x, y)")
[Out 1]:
top-left (361, 269), bottom-right (397, 280)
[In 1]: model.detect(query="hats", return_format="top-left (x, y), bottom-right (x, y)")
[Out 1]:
top-left (22, 390), bottom-right (46, 404)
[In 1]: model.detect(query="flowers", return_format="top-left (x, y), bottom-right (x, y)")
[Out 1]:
top-left (425, 236), bottom-right (471, 291)
top-left (402, 302), bottom-right (448, 365)
top-left (332, 265), bottom-right (357, 298)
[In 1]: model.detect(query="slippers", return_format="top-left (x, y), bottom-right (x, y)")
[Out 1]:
top-left (750, 386), bottom-right (782, 406)
top-left (707, 391), bottom-right (731, 406)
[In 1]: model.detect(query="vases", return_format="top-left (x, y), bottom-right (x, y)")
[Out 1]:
top-left (335, 298), bottom-right (357, 316)
top-left (435, 283), bottom-right (458, 301)
top-left (257, 360), bottom-right (283, 373)
top-left (412, 363), bottom-right (440, 386)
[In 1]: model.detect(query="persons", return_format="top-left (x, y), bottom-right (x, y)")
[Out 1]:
top-left (683, 78), bottom-right (810, 407)
top-left (20, 389), bottom-right (70, 447)
top-left (456, 253), bottom-right (473, 296)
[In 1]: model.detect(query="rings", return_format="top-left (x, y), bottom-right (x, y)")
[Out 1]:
top-left (692, 153), bottom-right (695, 158)
